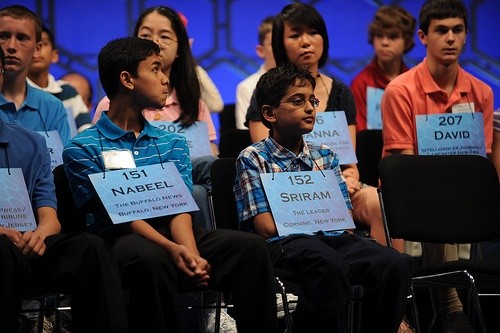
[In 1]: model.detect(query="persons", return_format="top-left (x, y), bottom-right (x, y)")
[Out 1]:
top-left (233, 4), bottom-right (410, 333)
top-left (0, 5), bottom-right (129, 333)
top-left (349, 0), bottom-right (500, 333)
top-left (62, 6), bottom-right (277, 333)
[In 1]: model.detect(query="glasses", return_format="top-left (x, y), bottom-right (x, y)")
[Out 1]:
top-left (138, 33), bottom-right (178, 48)
top-left (272, 97), bottom-right (319, 107)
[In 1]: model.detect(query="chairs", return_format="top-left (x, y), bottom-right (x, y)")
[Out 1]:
top-left (20, 104), bottom-right (500, 333)
